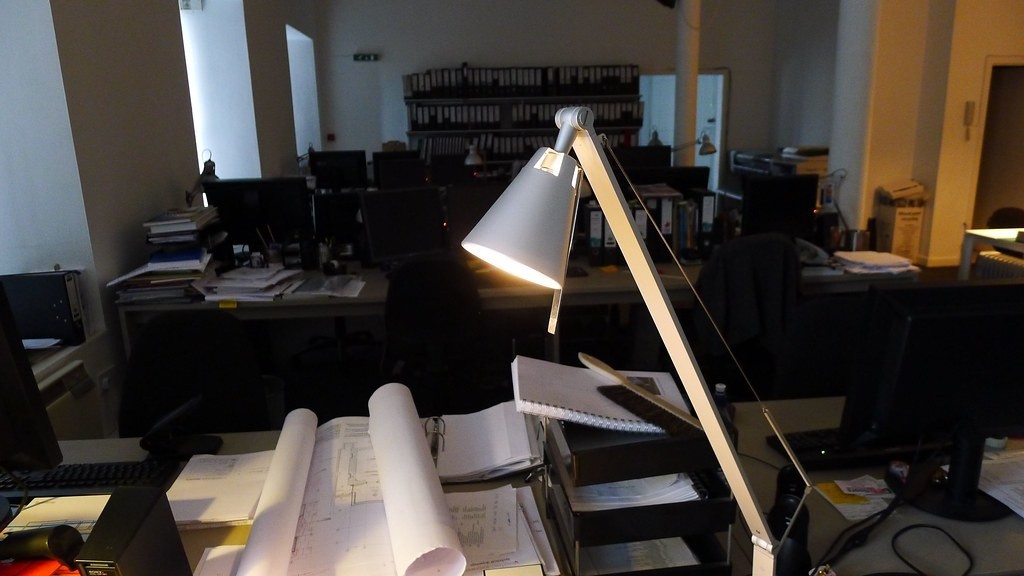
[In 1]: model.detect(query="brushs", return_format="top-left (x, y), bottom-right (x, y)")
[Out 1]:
top-left (578, 352), bottom-right (706, 440)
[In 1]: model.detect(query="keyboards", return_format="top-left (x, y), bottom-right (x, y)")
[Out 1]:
top-left (767, 427), bottom-right (950, 471)
top-left (0, 459), bottom-right (176, 503)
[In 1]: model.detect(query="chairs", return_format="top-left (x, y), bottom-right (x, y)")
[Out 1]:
top-left (201, 146), bottom-right (709, 281)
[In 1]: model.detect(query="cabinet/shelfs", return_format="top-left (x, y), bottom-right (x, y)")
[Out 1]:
top-left (405, 92), bottom-right (641, 167)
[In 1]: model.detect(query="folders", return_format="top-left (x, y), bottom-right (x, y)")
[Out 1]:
top-left (412, 64), bottom-right (640, 96)
top-left (584, 198), bottom-right (672, 266)
top-left (468, 134), bottom-right (624, 157)
top-left (408, 103), bottom-right (646, 129)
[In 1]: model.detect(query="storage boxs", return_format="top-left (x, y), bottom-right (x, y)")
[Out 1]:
top-left (875, 180), bottom-right (925, 262)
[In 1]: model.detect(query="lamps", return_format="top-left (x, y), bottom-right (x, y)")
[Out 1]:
top-left (461, 106), bottom-right (815, 576)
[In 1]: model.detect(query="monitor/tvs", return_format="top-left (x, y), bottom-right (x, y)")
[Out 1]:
top-left (0, 280), bottom-right (64, 522)
top-left (202, 150), bottom-right (493, 282)
top-left (837, 278), bottom-right (1024, 522)
top-left (740, 171), bottom-right (819, 245)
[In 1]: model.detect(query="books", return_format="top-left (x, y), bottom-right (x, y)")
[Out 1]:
top-left (511, 355), bottom-right (690, 434)
top-left (166, 450), bottom-right (274, 531)
top-left (118, 205), bottom-right (366, 304)
top-left (728, 146), bottom-right (829, 175)
top-left (420, 136), bottom-right (465, 164)
top-left (630, 183), bottom-right (682, 196)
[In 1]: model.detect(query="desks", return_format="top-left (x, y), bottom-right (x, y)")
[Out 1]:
top-left (313, 164), bottom-right (504, 205)
top-left (117, 259), bottom-right (1024, 435)
top-left (27, 329), bottom-right (116, 442)
top-left (54, 397), bottom-right (1024, 576)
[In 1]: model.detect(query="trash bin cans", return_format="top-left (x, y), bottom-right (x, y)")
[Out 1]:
top-left (875, 198), bottom-right (924, 264)
